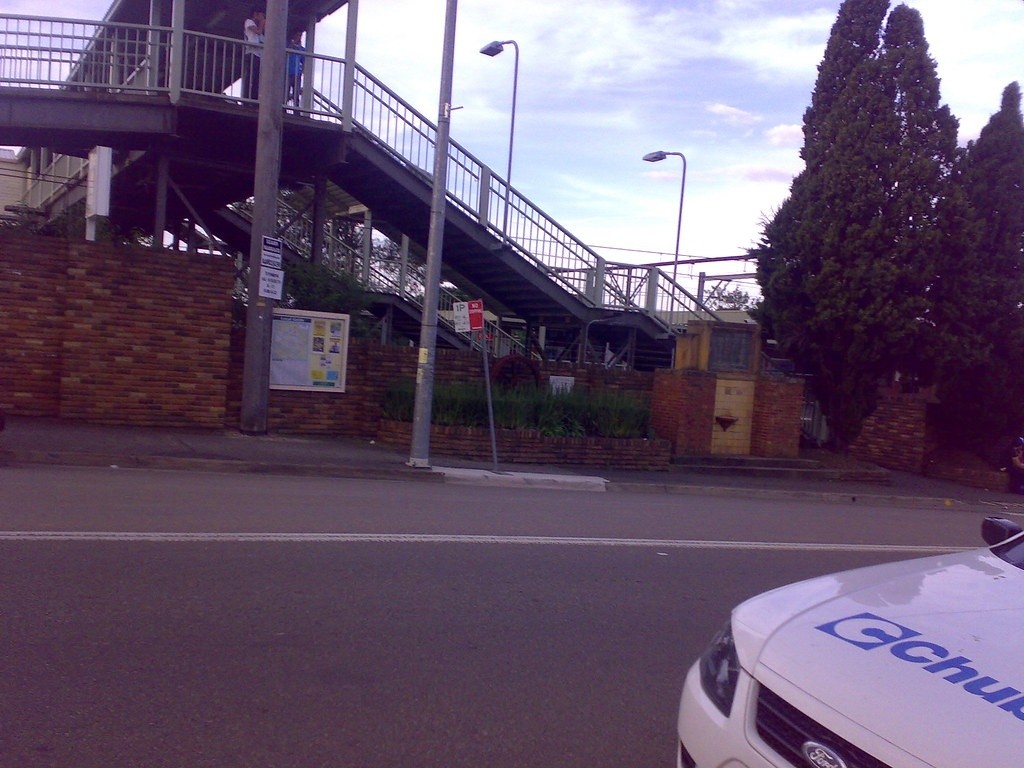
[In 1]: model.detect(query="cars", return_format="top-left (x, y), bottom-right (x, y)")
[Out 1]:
top-left (676, 516), bottom-right (1024, 768)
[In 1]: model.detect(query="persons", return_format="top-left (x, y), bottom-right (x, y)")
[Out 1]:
top-left (282, 26), bottom-right (305, 115)
top-left (241, 8), bottom-right (266, 108)
top-left (1004, 435), bottom-right (1024, 495)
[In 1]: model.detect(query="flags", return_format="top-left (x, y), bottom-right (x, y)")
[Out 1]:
top-left (605, 350), bottom-right (627, 369)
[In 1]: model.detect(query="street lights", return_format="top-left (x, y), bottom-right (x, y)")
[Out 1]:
top-left (643, 151), bottom-right (686, 333)
top-left (480, 40), bottom-right (519, 244)
